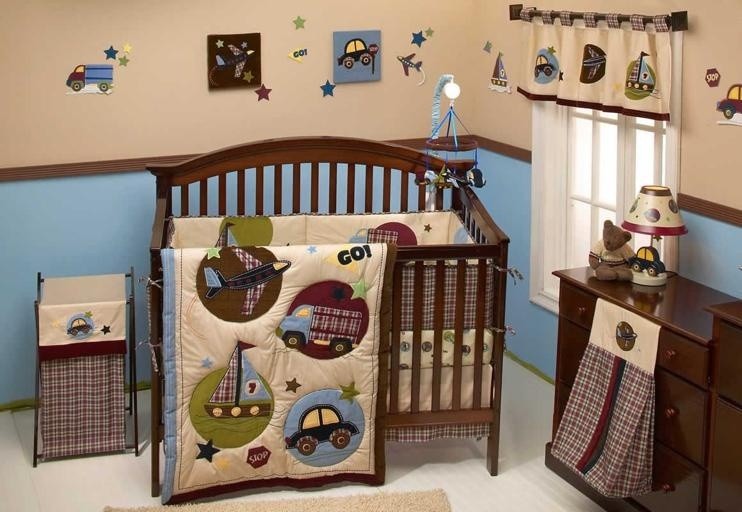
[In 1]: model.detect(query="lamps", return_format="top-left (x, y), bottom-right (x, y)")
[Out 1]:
top-left (418, 73), bottom-right (486, 194)
top-left (622, 183), bottom-right (688, 288)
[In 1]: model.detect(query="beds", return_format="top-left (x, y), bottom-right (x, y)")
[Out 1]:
top-left (141, 136), bottom-right (511, 498)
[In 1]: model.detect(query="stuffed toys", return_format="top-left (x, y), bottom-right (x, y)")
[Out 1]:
top-left (587, 220), bottom-right (636, 282)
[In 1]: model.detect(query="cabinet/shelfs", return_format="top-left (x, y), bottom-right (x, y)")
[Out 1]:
top-left (543, 264), bottom-right (741, 512)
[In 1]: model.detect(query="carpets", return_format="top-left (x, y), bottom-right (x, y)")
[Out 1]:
top-left (103, 486), bottom-right (454, 512)
